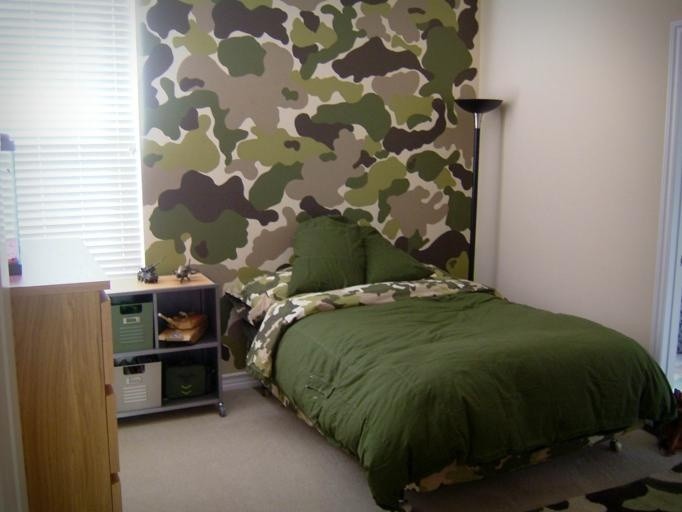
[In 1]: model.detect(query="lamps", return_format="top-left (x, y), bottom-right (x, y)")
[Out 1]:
top-left (453, 98), bottom-right (503, 281)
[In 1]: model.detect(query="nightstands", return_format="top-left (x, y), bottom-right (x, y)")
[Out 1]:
top-left (104, 272), bottom-right (226, 419)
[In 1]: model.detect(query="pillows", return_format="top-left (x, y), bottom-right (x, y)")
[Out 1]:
top-left (359, 226), bottom-right (424, 283)
top-left (285, 215), bottom-right (367, 298)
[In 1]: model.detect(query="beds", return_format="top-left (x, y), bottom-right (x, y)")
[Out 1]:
top-left (220, 264), bottom-right (681, 512)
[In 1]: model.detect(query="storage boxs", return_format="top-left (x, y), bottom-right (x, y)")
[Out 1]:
top-left (114, 362), bottom-right (161, 412)
top-left (165, 368), bottom-right (206, 400)
top-left (112, 302), bottom-right (154, 353)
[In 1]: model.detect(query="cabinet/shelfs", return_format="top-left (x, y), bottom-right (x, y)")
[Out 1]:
top-left (10, 262), bottom-right (123, 511)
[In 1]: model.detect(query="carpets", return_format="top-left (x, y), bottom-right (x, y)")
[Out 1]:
top-left (524, 462), bottom-right (682, 512)
